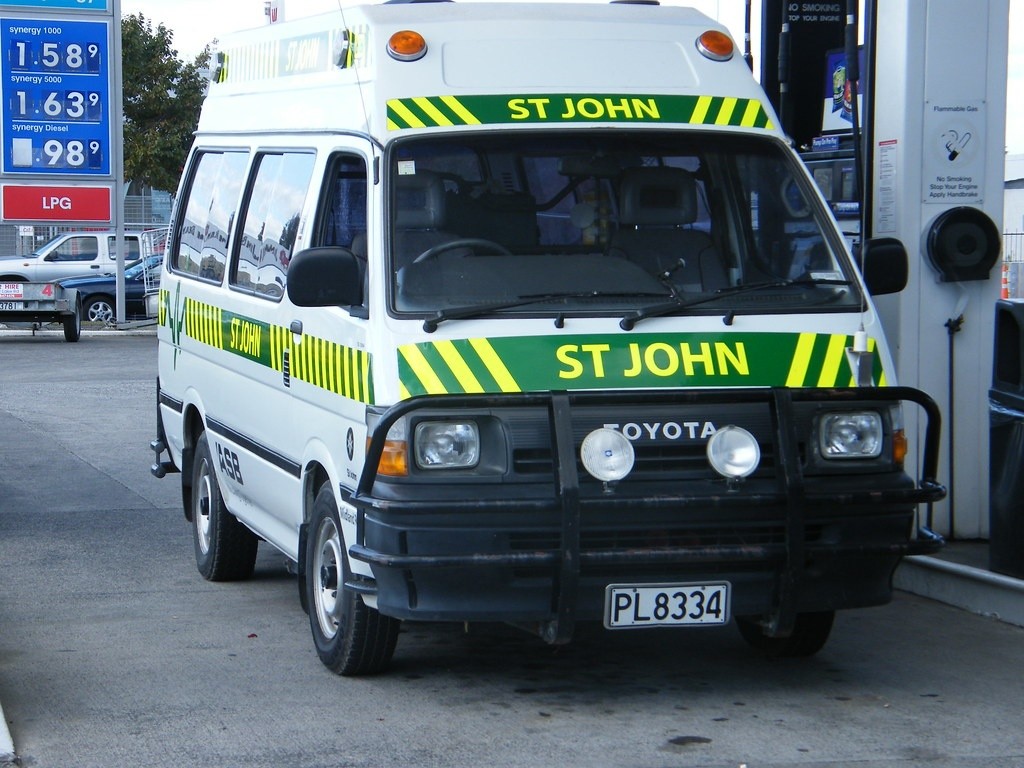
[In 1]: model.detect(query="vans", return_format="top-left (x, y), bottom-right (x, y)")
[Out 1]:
top-left (150, 1), bottom-right (948, 679)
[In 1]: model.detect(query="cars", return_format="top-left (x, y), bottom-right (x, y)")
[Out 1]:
top-left (49, 252), bottom-right (165, 323)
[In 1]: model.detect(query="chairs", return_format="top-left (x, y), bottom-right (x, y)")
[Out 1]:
top-left (441, 189), bottom-right (540, 253)
top-left (612, 167), bottom-right (728, 294)
top-left (352, 168), bottom-right (475, 301)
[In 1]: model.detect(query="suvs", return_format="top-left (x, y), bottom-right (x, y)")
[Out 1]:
top-left (0, 229), bottom-right (166, 286)
